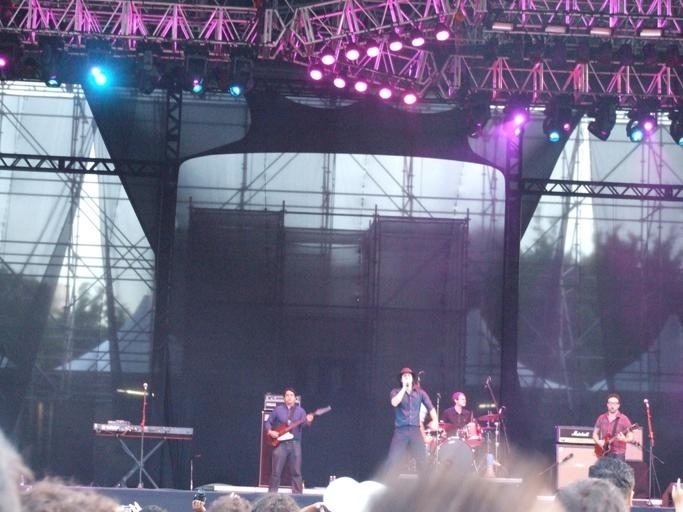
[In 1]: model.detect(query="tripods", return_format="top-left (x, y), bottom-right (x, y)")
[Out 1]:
top-left (472, 384), bottom-right (511, 475)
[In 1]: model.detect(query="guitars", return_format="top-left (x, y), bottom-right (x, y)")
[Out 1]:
top-left (595, 422), bottom-right (640, 459)
top-left (265, 405), bottom-right (338, 445)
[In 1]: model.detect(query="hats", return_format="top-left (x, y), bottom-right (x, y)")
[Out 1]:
top-left (397, 368), bottom-right (418, 384)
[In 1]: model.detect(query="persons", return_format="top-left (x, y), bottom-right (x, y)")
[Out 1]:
top-left (592, 391), bottom-right (634, 463)
top-left (441, 389), bottom-right (480, 443)
top-left (385, 368), bottom-right (438, 483)
top-left (3, 433), bottom-right (683, 512)
top-left (264, 386), bottom-right (315, 494)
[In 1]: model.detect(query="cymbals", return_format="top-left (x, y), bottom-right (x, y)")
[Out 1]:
top-left (480, 426), bottom-right (497, 430)
top-left (439, 421), bottom-right (455, 431)
top-left (477, 413), bottom-right (499, 422)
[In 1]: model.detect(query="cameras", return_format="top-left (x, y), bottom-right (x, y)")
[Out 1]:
top-left (192, 493), bottom-right (207, 503)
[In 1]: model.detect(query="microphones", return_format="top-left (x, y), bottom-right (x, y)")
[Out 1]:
top-left (484, 377), bottom-right (490, 389)
top-left (143, 382), bottom-right (148, 395)
top-left (563, 452), bottom-right (574, 462)
top-left (643, 398), bottom-right (650, 408)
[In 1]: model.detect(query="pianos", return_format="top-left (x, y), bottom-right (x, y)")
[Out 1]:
top-left (90, 421), bottom-right (193, 439)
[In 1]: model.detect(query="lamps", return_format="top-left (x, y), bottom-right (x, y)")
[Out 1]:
top-left (320, 23), bottom-right (449, 65)
top-left (482, 34), bottom-right (681, 67)
top-left (464, 92), bottom-right (682, 145)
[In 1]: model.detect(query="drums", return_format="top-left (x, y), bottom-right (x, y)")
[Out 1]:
top-left (429, 434), bottom-right (474, 470)
top-left (467, 423), bottom-right (485, 447)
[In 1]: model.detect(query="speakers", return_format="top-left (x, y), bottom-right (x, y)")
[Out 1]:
top-left (553, 443), bottom-right (599, 492)
top-left (258, 411), bottom-right (292, 488)
